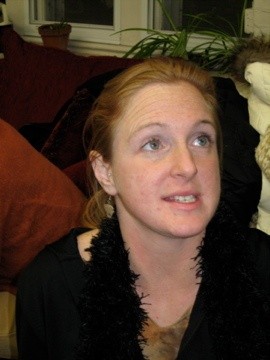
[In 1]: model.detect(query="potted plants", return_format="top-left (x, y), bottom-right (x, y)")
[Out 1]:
top-left (39, 17), bottom-right (71, 50)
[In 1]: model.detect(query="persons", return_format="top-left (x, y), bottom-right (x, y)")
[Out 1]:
top-left (0, 116), bottom-right (89, 360)
top-left (14, 57), bottom-right (270, 360)
